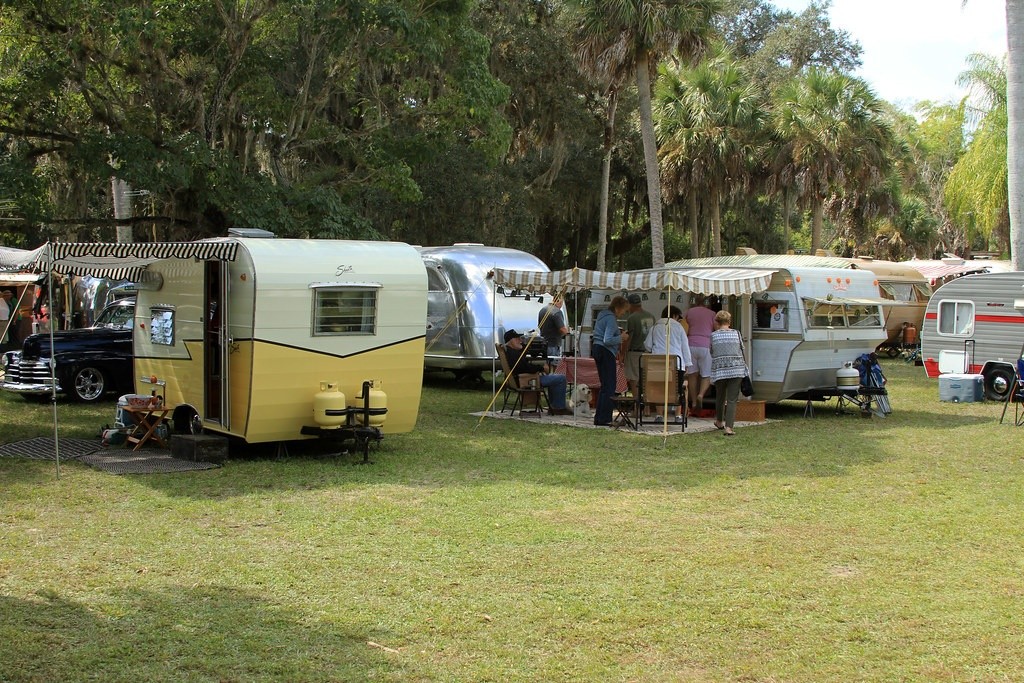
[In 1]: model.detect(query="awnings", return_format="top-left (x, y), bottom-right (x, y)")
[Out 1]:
top-left (664, 254), bottom-right (852, 270)
top-left (0, 243), bottom-right (239, 479)
top-left (491, 265), bottom-right (779, 438)
top-left (902, 263), bottom-right (988, 288)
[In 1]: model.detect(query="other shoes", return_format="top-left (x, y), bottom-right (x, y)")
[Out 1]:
top-left (653, 416), bottom-right (663, 422)
top-left (594, 421), bottom-right (614, 425)
top-left (553, 408), bottom-right (574, 415)
top-left (694, 397), bottom-right (702, 417)
top-left (675, 416), bottom-right (683, 422)
top-left (548, 408), bottom-right (553, 416)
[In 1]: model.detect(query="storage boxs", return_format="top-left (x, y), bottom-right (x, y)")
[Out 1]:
top-left (938, 373), bottom-right (984, 403)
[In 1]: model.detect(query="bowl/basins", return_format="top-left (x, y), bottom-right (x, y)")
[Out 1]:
top-left (124, 395), bottom-right (154, 409)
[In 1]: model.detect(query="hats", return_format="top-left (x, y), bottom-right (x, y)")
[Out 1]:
top-left (3, 290), bottom-right (14, 295)
top-left (628, 294), bottom-right (640, 303)
top-left (504, 329), bottom-right (524, 344)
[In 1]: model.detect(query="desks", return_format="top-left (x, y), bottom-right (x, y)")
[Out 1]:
top-left (554, 356), bottom-right (628, 399)
top-left (121, 405), bottom-right (175, 451)
top-left (610, 397), bottom-right (636, 431)
top-left (516, 387), bottom-right (544, 418)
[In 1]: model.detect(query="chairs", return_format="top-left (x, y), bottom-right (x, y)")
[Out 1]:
top-left (636, 354), bottom-right (687, 432)
top-left (495, 343), bottom-right (555, 416)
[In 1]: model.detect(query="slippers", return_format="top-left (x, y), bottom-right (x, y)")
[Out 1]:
top-left (714, 421), bottom-right (724, 430)
top-left (723, 430), bottom-right (735, 436)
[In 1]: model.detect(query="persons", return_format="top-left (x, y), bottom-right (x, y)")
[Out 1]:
top-left (626, 293), bottom-right (656, 398)
top-left (538, 293), bottom-right (570, 356)
top-left (498, 318), bottom-right (507, 345)
top-left (683, 294), bottom-right (720, 410)
top-left (709, 309), bottom-right (750, 436)
top-left (504, 329), bottom-right (574, 415)
top-left (643, 304), bottom-right (693, 422)
top-left (592, 295), bottom-right (631, 426)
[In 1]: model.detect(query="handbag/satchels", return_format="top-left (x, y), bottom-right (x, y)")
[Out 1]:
top-left (677, 317), bottom-right (689, 335)
top-left (740, 376), bottom-right (754, 397)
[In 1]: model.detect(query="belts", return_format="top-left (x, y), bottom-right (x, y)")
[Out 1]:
top-left (628, 349), bottom-right (646, 352)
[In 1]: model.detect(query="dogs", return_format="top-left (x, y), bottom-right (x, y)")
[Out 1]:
top-left (566, 384), bottom-right (597, 419)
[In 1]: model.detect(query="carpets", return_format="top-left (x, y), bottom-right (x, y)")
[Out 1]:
top-left (76, 447), bottom-right (221, 475)
top-left (467, 407), bottom-right (785, 436)
top-left (0, 437), bottom-right (112, 461)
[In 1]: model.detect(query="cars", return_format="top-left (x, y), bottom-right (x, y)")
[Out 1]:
top-left (0, 295), bottom-right (174, 405)
